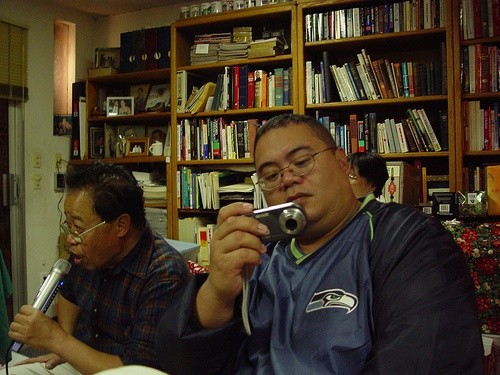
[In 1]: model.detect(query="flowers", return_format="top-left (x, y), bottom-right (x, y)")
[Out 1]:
top-left (440, 217), bottom-right (500, 334)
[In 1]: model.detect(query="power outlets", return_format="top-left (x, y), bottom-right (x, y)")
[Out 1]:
top-left (32, 152), bottom-right (41, 168)
top-left (55, 153), bottom-right (62, 167)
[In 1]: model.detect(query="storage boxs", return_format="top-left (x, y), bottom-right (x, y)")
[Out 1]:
top-left (88, 68), bottom-right (117, 79)
top-left (165, 238), bottom-right (201, 263)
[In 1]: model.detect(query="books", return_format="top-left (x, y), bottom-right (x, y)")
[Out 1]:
top-left (135, 165), bottom-right (268, 267)
top-left (177, 0), bottom-right (500, 160)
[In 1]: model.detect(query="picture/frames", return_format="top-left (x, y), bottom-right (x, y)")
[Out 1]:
top-left (125, 137), bottom-right (147, 157)
top-left (107, 96), bottom-right (134, 116)
top-left (94, 47), bottom-right (120, 69)
top-left (130, 83), bottom-right (150, 112)
top-left (90, 127), bottom-right (105, 158)
top-left (144, 83), bottom-right (171, 112)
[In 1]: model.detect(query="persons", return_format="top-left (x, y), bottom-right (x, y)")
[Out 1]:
top-left (135, 88), bottom-right (145, 109)
top-left (346, 151), bottom-right (389, 203)
top-left (153, 115), bottom-right (484, 375)
top-left (133, 144), bottom-right (141, 153)
top-left (95, 136), bottom-right (104, 154)
top-left (109, 100), bottom-right (130, 115)
top-left (8, 161), bottom-right (192, 375)
top-left (150, 87), bottom-right (166, 109)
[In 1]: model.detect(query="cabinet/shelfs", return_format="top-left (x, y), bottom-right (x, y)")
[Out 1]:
top-left (85, 0), bottom-right (500, 267)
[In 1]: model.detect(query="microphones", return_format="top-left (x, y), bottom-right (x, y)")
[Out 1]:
top-left (13, 258), bottom-right (72, 352)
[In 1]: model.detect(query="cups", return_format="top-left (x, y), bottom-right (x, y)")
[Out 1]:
top-left (149, 142), bottom-right (163, 156)
top-left (179, 0), bottom-right (284, 19)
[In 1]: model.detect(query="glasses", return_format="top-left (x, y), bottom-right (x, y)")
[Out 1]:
top-left (59, 220), bottom-right (108, 244)
top-left (348, 174), bottom-right (359, 180)
top-left (255, 146), bottom-right (336, 192)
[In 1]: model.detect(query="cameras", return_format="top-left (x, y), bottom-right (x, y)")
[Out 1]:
top-left (238, 202), bottom-right (309, 245)
